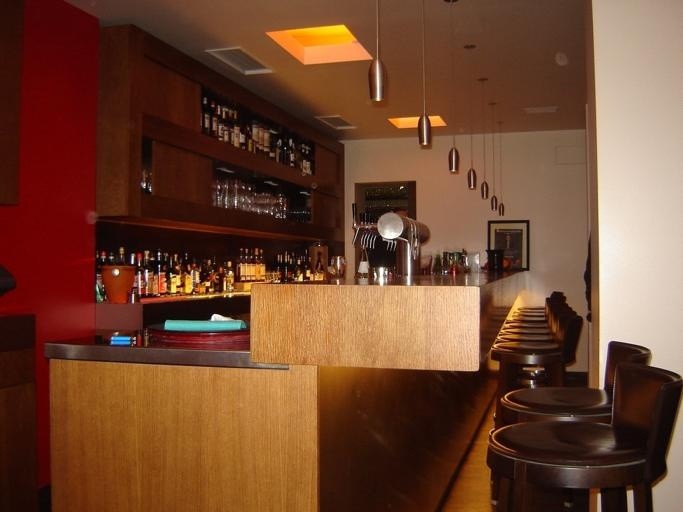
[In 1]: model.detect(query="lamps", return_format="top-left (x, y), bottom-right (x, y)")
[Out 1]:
top-left (368, 0), bottom-right (505, 216)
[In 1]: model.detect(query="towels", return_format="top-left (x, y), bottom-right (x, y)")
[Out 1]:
top-left (165, 314), bottom-right (246, 332)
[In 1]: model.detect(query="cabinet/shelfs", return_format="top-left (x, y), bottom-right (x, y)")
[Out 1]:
top-left (98, 24), bottom-right (344, 302)
top-left (353, 181), bottom-right (416, 286)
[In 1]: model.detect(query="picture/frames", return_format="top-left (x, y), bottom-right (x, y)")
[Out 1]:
top-left (488, 220), bottom-right (530, 271)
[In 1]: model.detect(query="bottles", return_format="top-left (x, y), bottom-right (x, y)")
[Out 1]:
top-left (423, 248), bottom-right (470, 275)
top-left (485, 250), bottom-right (495, 275)
top-left (358, 277), bottom-right (369, 285)
top-left (212, 180), bottom-right (288, 220)
top-left (289, 207), bottom-right (311, 224)
top-left (357, 245), bottom-right (369, 277)
top-left (360, 200), bottom-right (378, 223)
top-left (96, 241), bottom-right (345, 304)
top-left (201, 97), bottom-right (312, 175)
top-left (370, 264), bottom-right (398, 278)
top-left (494, 249), bottom-right (504, 276)
